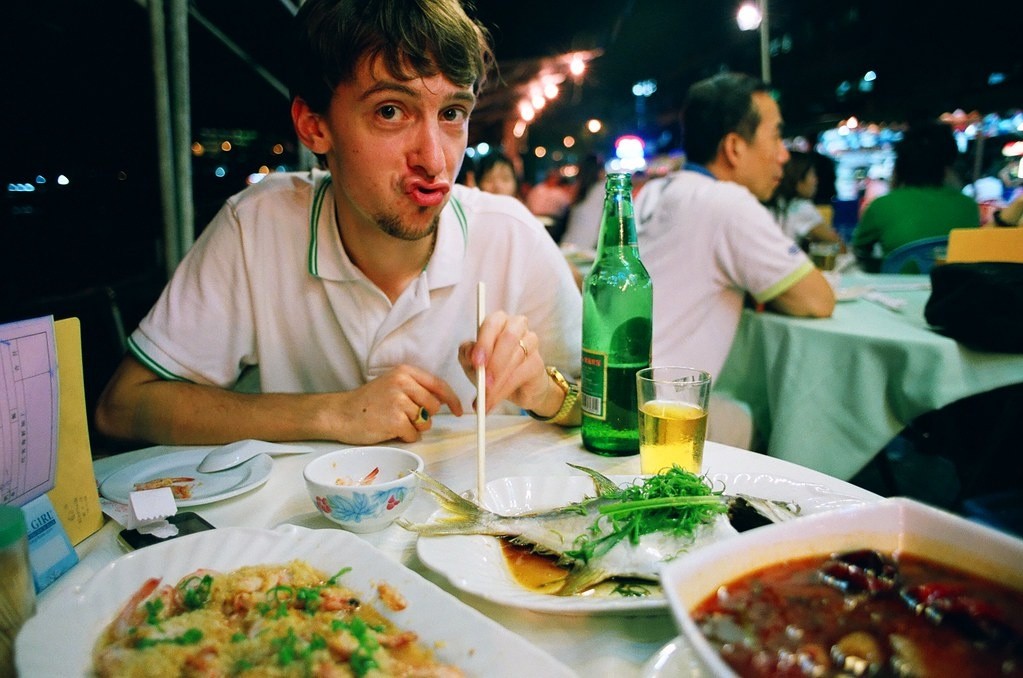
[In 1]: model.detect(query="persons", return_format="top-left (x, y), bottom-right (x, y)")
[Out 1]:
top-left (474, 153), bottom-right (518, 197)
top-left (96, 0), bottom-right (584, 445)
top-left (527, 155), bottom-right (610, 251)
top-left (851, 118), bottom-right (980, 273)
top-left (982, 195), bottom-right (1023, 227)
top-left (765, 154), bottom-right (840, 244)
top-left (631, 74), bottom-right (836, 447)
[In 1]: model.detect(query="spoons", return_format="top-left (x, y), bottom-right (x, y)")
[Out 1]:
top-left (198, 439), bottom-right (315, 473)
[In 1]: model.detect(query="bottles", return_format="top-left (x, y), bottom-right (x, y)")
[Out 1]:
top-left (581, 174), bottom-right (653, 457)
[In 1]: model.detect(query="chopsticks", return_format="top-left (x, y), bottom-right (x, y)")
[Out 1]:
top-left (477, 281), bottom-right (486, 508)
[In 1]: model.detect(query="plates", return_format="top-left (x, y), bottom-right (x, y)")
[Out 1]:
top-left (647, 635), bottom-right (716, 678)
top-left (14, 524), bottom-right (579, 678)
top-left (100, 448), bottom-right (274, 507)
top-left (417, 475), bottom-right (869, 616)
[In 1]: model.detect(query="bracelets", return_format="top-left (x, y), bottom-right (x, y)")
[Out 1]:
top-left (993, 211), bottom-right (1009, 226)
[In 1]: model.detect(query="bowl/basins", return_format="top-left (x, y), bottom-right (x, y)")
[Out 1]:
top-left (302, 447), bottom-right (425, 533)
top-left (661, 498), bottom-right (1023, 678)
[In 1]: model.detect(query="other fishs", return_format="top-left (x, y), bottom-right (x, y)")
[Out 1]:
top-left (394, 462), bottom-right (804, 597)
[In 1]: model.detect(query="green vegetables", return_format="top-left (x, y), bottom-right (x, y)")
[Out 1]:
top-left (551, 460), bottom-right (729, 597)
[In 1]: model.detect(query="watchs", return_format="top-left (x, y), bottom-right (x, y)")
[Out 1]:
top-left (526, 367), bottom-right (579, 423)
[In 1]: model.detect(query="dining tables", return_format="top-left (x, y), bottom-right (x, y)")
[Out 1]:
top-left (733, 270), bottom-right (1023, 482)
top-left (92, 423), bottom-right (1023, 678)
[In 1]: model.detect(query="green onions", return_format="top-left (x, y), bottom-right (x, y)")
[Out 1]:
top-left (123, 565), bottom-right (385, 678)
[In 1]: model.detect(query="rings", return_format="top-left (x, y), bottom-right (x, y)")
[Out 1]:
top-left (412, 407), bottom-right (429, 424)
top-left (520, 340), bottom-right (527, 356)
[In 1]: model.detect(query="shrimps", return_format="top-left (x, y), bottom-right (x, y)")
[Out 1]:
top-left (335, 467), bottom-right (379, 485)
top-left (133, 477), bottom-right (195, 492)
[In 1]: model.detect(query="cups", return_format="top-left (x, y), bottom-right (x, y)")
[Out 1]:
top-left (636, 366), bottom-right (712, 475)
top-left (809, 244), bottom-right (838, 273)
top-left (821, 273), bottom-right (841, 292)
